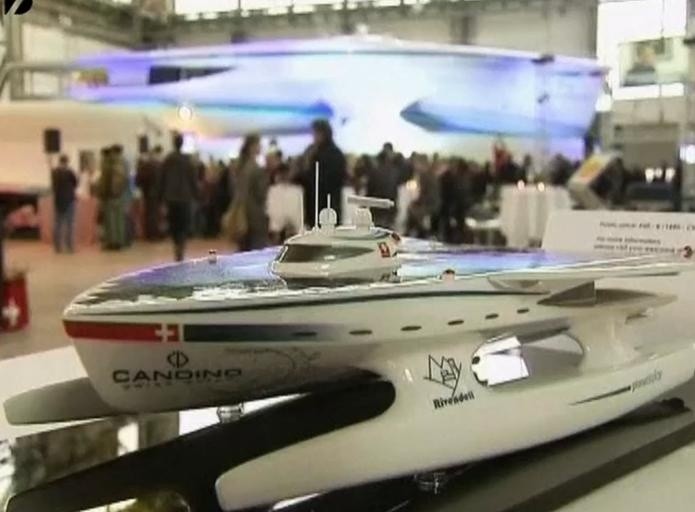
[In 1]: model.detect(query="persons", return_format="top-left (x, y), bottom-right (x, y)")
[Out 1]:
top-left (289, 118), bottom-right (348, 232)
top-left (623, 40), bottom-right (660, 87)
top-left (153, 132), bottom-right (200, 262)
top-left (51, 131), bottom-right (685, 255)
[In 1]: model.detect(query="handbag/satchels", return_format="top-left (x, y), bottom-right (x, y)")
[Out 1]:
top-left (221, 208), bottom-right (246, 239)
